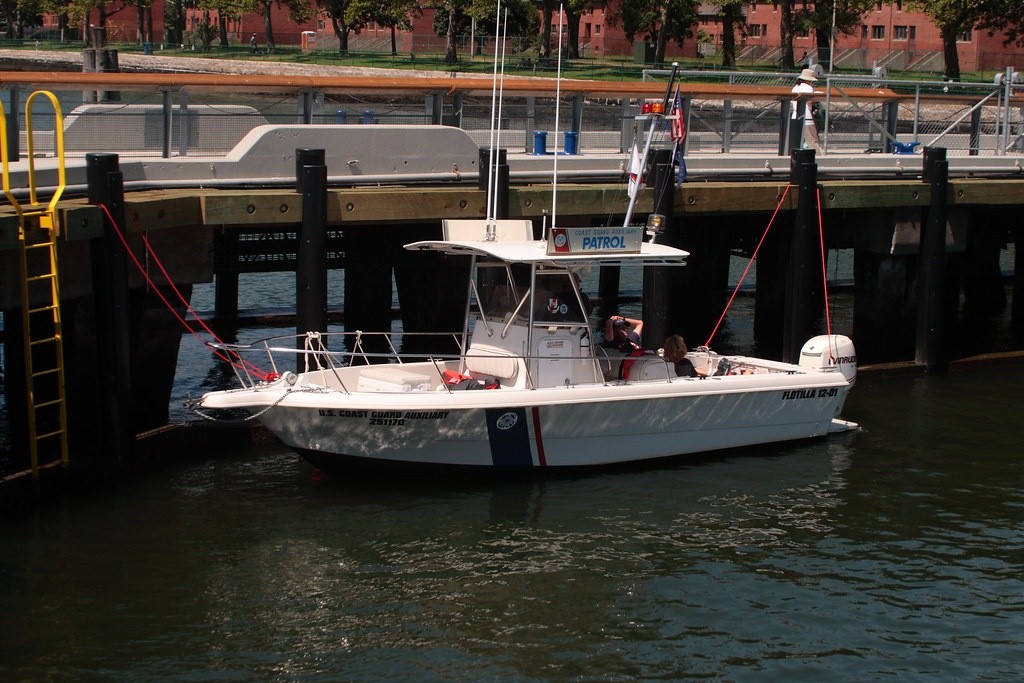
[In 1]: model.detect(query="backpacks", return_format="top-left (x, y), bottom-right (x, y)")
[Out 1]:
top-left (811, 101), bottom-right (832, 132)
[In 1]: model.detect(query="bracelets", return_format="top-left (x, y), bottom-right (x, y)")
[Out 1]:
top-left (622, 317), bottom-right (626, 321)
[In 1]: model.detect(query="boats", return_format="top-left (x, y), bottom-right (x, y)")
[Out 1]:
top-left (196, 1), bottom-right (869, 470)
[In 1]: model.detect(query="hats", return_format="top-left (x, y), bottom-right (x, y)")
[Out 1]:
top-left (798, 69), bottom-right (818, 82)
top-left (612, 319), bottom-right (631, 329)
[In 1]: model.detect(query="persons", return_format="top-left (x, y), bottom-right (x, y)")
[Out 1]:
top-left (791, 68), bottom-right (825, 156)
top-left (604, 315), bottom-right (754, 378)
top-left (548, 273), bottom-right (593, 320)
